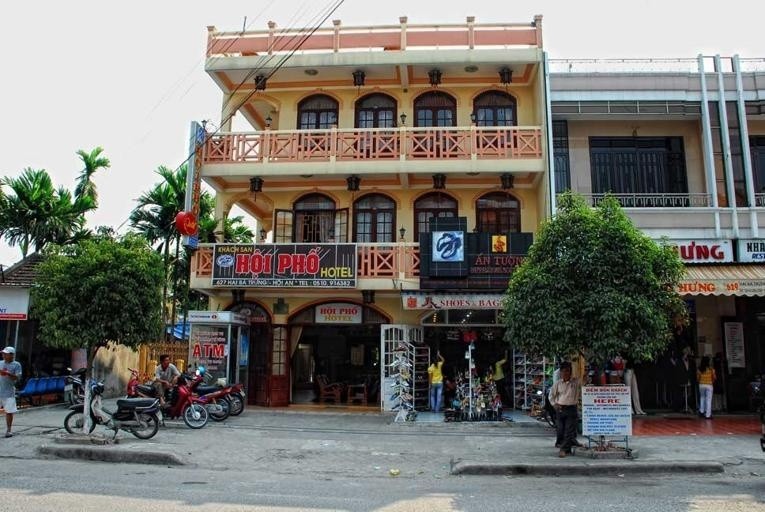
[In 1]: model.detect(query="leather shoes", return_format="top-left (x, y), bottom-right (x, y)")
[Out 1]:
top-left (160, 402), bottom-right (170, 409)
top-left (559, 450), bottom-right (565, 458)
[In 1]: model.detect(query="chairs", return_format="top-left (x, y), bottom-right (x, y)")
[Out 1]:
top-left (14, 373), bottom-right (65, 409)
top-left (315, 364), bottom-right (375, 404)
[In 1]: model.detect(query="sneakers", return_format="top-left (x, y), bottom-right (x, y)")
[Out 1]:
top-left (5, 432), bottom-right (12, 437)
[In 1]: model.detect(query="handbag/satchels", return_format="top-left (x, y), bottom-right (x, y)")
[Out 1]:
top-left (127, 369), bottom-right (140, 397)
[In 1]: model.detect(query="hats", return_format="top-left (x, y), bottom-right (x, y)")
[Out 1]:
top-left (0, 347), bottom-right (15, 354)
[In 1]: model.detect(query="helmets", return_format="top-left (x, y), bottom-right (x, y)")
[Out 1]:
top-left (197, 366), bottom-right (205, 375)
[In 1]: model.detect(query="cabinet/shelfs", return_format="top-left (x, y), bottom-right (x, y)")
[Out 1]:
top-left (510, 336), bottom-right (558, 413)
top-left (443, 364), bottom-right (502, 423)
top-left (407, 338), bottom-right (431, 412)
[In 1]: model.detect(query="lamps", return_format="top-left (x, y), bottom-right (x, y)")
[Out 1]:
top-left (400, 223), bottom-right (406, 239)
top-left (198, 111), bottom-right (482, 131)
top-left (328, 225), bottom-right (336, 239)
top-left (257, 226), bottom-right (266, 240)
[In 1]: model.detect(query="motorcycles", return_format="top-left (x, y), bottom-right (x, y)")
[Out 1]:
top-left (126, 367), bottom-right (148, 399)
top-left (64, 378), bottom-right (164, 444)
top-left (181, 364), bottom-right (246, 422)
top-left (134, 374), bottom-right (209, 430)
top-left (536, 385), bottom-right (558, 429)
top-left (64, 366), bottom-right (106, 405)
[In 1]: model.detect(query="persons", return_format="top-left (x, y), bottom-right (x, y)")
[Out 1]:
top-left (0, 346), bottom-right (23, 437)
top-left (425, 348), bottom-right (446, 414)
top-left (486, 349), bottom-right (509, 398)
top-left (548, 359), bottom-right (590, 457)
top-left (675, 345), bottom-right (696, 381)
top-left (624, 359), bottom-right (649, 418)
top-left (698, 356), bottom-right (718, 419)
top-left (155, 354), bottom-right (180, 407)
top-left (610, 355), bottom-right (624, 383)
